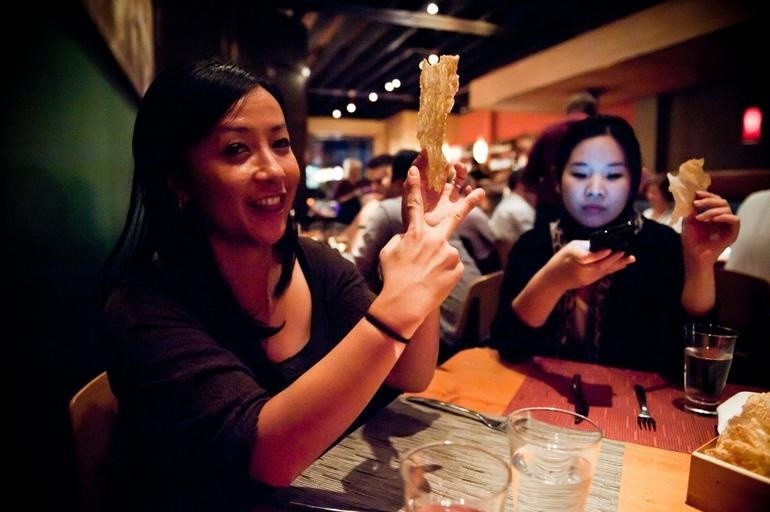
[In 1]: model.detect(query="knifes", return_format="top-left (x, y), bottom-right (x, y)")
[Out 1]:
top-left (573, 372), bottom-right (589, 424)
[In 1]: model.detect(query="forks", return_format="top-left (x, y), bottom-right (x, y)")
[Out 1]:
top-left (406, 396), bottom-right (528, 432)
top-left (634, 384), bottom-right (655, 433)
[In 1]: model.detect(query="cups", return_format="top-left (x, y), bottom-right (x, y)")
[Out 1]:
top-left (683, 324), bottom-right (739, 414)
top-left (400, 407), bottom-right (604, 512)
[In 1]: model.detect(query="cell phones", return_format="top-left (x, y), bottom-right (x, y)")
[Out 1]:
top-left (589, 219), bottom-right (633, 257)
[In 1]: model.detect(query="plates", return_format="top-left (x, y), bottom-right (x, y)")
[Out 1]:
top-left (716, 388), bottom-right (761, 435)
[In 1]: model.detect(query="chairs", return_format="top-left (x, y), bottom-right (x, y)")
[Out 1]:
top-left (66, 369), bottom-right (119, 512)
top-left (446, 268), bottom-right (506, 353)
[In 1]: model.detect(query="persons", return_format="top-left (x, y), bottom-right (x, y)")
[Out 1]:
top-left (100, 59), bottom-right (481, 489)
top-left (521, 91), bottom-right (600, 228)
top-left (332, 147), bottom-right (540, 363)
top-left (643, 177), bottom-right (682, 231)
top-left (724, 190), bottom-right (770, 280)
top-left (489, 112), bottom-right (741, 364)
